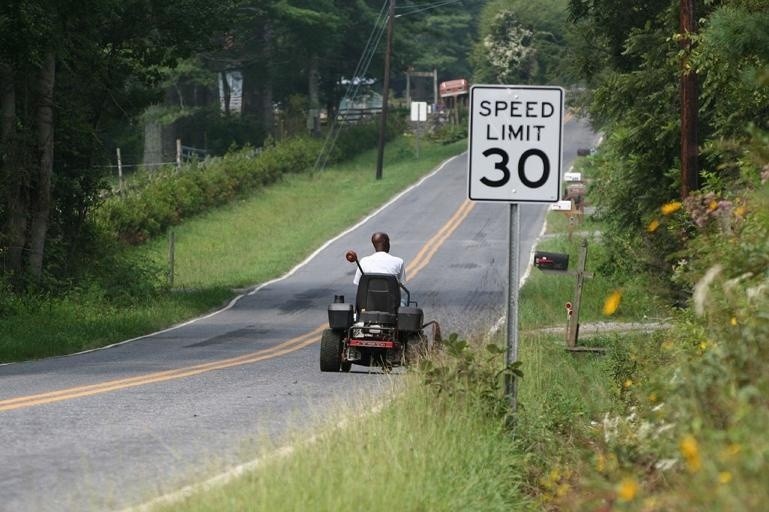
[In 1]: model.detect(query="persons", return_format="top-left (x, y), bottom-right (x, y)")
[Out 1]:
top-left (351, 231), bottom-right (408, 307)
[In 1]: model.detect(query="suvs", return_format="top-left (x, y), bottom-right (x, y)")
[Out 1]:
top-left (317, 270), bottom-right (430, 374)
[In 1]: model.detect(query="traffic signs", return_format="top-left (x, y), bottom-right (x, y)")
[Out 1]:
top-left (468, 84), bottom-right (564, 204)
